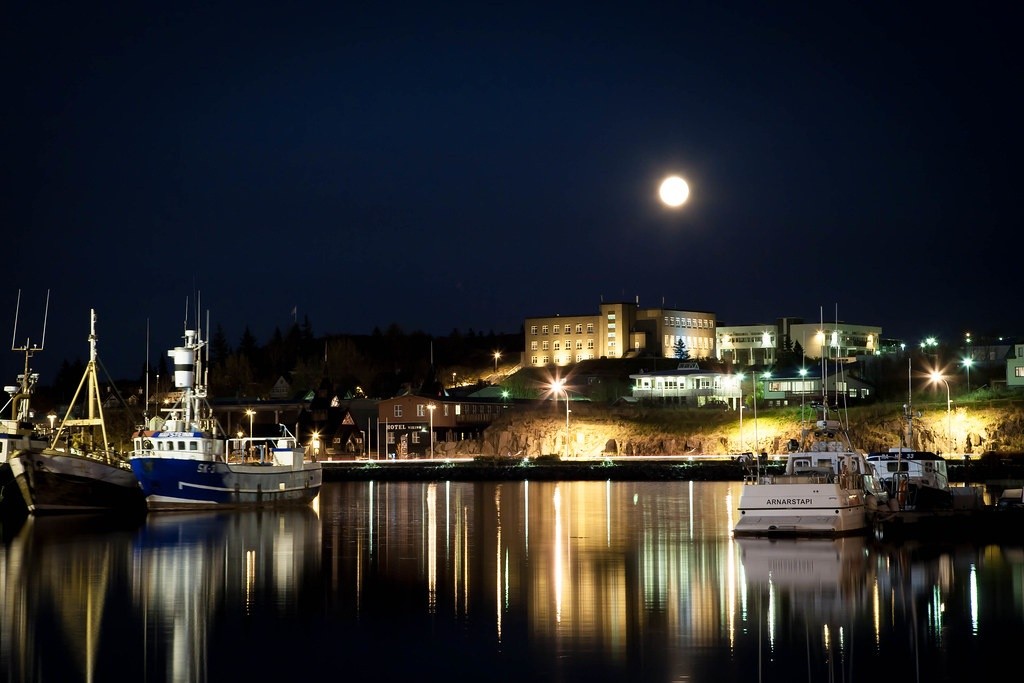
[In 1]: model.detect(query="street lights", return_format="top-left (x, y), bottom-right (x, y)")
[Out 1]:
top-left (310, 430), bottom-right (320, 461)
top-left (245, 409), bottom-right (256, 462)
top-left (547, 381), bottom-right (573, 459)
top-left (424, 404), bottom-right (437, 459)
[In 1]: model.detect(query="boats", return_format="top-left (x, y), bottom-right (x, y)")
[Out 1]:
top-left (863, 358), bottom-right (948, 498)
top-left (0, 293), bottom-right (63, 511)
top-left (9, 306), bottom-right (151, 516)
top-left (730, 302), bottom-right (876, 540)
top-left (864, 477), bottom-right (1023, 539)
top-left (130, 288), bottom-right (326, 511)
